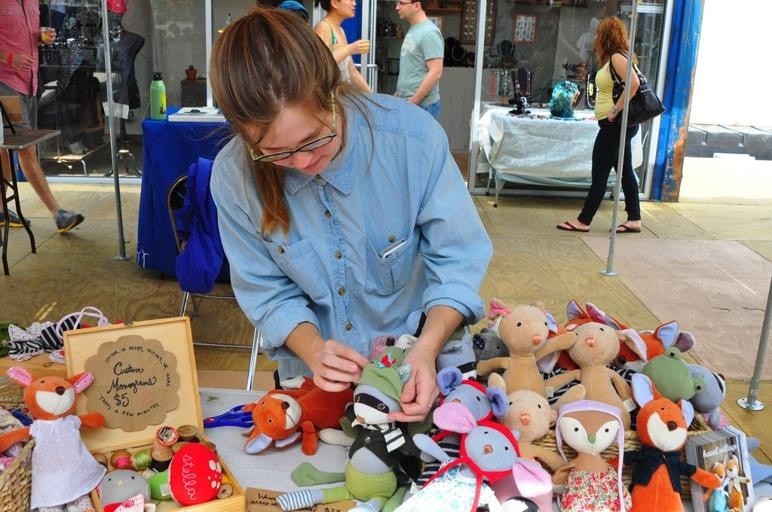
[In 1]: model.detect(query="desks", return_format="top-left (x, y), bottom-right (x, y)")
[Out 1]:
top-left (1, 126), bottom-right (63, 276)
top-left (199, 387), bottom-right (370, 512)
top-left (482, 101), bottom-right (630, 211)
top-left (140, 104), bottom-right (242, 284)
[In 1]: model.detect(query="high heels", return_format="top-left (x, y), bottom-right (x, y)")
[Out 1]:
top-left (75, 139), bottom-right (89, 152)
top-left (64, 139), bottom-right (84, 154)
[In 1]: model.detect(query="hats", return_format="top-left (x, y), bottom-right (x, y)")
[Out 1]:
top-left (106, 0), bottom-right (127, 14)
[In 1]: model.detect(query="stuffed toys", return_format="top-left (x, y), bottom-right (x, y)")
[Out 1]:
top-left (1, 369), bottom-right (223, 512)
top-left (243, 301), bottom-right (749, 511)
top-left (0, 300), bottom-right (772, 512)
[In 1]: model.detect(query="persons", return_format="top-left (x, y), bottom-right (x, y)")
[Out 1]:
top-left (308, 0), bottom-right (374, 92)
top-left (555, 17), bottom-right (642, 232)
top-left (209, 7), bottom-right (494, 423)
top-left (1, 1), bottom-right (86, 235)
top-left (392, 0), bottom-right (444, 126)
top-left (587, 3), bottom-right (608, 41)
top-left (532, 13), bottom-right (585, 104)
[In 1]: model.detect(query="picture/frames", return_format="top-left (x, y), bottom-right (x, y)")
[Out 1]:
top-left (512, 13), bottom-right (539, 44)
top-left (459, 1), bottom-right (497, 46)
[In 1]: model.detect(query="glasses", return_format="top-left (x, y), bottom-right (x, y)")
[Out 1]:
top-left (247, 93), bottom-right (337, 163)
top-left (395, 0), bottom-right (413, 5)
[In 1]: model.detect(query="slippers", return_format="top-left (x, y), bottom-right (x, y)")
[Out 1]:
top-left (617, 224), bottom-right (642, 234)
top-left (557, 221), bottom-right (590, 233)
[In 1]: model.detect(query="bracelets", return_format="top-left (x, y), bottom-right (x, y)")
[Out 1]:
top-left (7, 51), bottom-right (14, 68)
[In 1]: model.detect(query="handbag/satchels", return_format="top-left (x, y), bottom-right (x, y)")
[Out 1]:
top-left (611, 74), bottom-right (665, 128)
top-left (57, 79), bottom-right (89, 104)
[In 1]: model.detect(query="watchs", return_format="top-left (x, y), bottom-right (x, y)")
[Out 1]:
top-left (611, 106), bottom-right (619, 115)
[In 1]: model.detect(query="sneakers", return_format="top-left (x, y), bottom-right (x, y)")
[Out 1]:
top-left (0, 209), bottom-right (30, 227)
top-left (55, 209), bottom-right (84, 234)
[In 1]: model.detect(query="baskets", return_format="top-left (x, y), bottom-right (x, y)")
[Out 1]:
top-left (530, 412), bottom-right (713, 502)
top-left (0, 397), bottom-right (35, 512)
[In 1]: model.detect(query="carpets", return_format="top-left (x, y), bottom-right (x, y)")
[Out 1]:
top-left (578, 235), bottom-right (771, 383)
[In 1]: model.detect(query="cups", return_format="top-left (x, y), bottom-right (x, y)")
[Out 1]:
top-left (41, 26), bottom-right (52, 41)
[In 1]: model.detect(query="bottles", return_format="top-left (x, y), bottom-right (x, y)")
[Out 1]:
top-left (149, 71), bottom-right (167, 120)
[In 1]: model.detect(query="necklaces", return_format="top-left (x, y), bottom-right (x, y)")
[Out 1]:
top-left (452, 39), bottom-right (532, 107)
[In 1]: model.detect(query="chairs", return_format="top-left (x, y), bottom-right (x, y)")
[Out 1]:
top-left (165, 170), bottom-right (282, 390)
